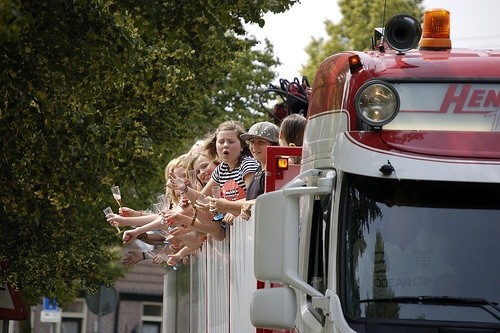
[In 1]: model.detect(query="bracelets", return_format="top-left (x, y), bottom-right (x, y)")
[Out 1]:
top-left (180, 186), bottom-right (188, 194)
top-left (191, 213), bottom-right (197, 227)
top-left (143, 252), bottom-right (146, 260)
top-left (140, 211), bottom-right (143, 216)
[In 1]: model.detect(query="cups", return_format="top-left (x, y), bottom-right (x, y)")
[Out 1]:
top-left (211, 186), bottom-right (220, 199)
top-left (198, 194), bottom-right (209, 204)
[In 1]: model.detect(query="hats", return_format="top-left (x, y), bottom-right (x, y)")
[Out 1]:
top-left (239, 121), bottom-right (280, 143)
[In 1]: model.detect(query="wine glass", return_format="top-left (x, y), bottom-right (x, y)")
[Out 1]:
top-left (103, 207), bottom-right (124, 235)
top-left (111, 186), bottom-right (128, 215)
top-left (154, 194), bottom-right (170, 246)
top-left (188, 170), bottom-right (197, 191)
top-left (158, 244), bottom-right (182, 270)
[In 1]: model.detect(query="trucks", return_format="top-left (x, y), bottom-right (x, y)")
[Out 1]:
top-left (160, 8), bottom-right (500, 332)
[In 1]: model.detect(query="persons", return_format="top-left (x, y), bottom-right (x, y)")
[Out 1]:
top-left (352, 178), bottom-right (458, 305)
top-left (278, 114), bottom-right (306, 165)
top-left (106, 119), bottom-right (260, 267)
top-left (240, 121), bottom-right (280, 221)
